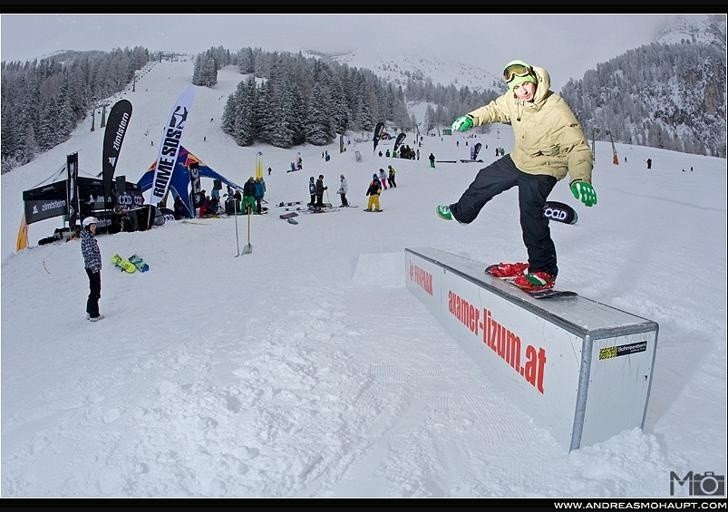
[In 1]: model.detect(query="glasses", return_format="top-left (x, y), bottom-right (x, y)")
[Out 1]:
top-left (501, 64), bottom-right (530, 82)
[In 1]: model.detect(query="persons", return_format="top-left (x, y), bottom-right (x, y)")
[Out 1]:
top-left (198, 131), bottom-right (694, 219)
top-left (80, 216), bottom-right (105, 322)
top-left (434, 59), bottom-right (598, 295)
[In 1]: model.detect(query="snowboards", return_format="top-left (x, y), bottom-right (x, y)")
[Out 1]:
top-left (364, 208), bottom-right (383, 212)
top-left (112, 254), bottom-right (135, 275)
top-left (485, 262), bottom-right (579, 298)
top-left (281, 199), bottom-right (357, 225)
top-left (128, 252), bottom-right (148, 273)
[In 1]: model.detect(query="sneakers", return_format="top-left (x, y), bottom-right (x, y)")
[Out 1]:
top-left (526, 274), bottom-right (549, 286)
top-left (436, 205), bottom-right (452, 221)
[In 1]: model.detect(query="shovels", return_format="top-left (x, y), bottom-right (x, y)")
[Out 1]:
top-left (242, 206), bottom-right (252, 255)
top-left (232, 198), bottom-right (240, 258)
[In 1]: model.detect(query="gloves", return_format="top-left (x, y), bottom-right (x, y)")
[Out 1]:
top-left (570, 181), bottom-right (597, 207)
top-left (450, 115), bottom-right (474, 133)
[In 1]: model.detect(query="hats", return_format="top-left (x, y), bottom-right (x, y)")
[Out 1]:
top-left (504, 60), bottom-right (536, 93)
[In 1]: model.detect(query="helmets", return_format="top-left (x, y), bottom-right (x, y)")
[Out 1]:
top-left (82, 216), bottom-right (99, 228)
